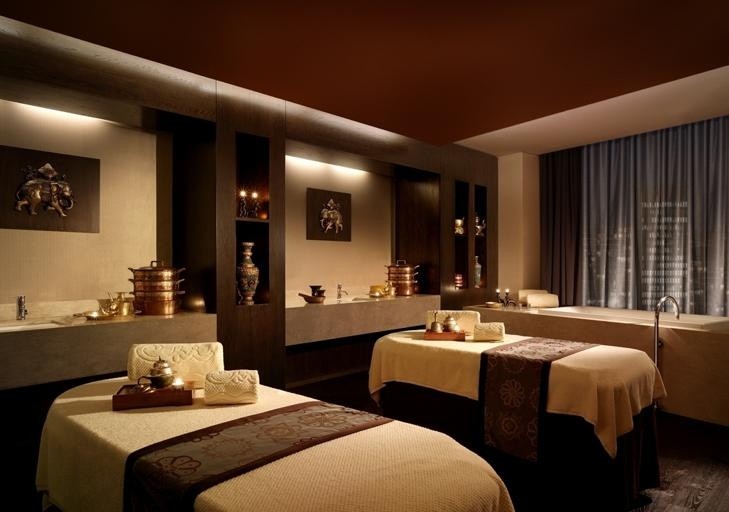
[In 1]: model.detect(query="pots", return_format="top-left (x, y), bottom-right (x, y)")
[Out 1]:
top-left (127, 261), bottom-right (187, 316)
top-left (384, 260), bottom-right (419, 296)
top-left (97, 297), bottom-right (136, 317)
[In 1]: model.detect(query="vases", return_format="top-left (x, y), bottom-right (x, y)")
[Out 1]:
top-left (473, 256), bottom-right (482, 288)
top-left (236, 241), bottom-right (259, 306)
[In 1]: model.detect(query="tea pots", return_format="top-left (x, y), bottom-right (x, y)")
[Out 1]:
top-left (137, 355), bottom-right (175, 390)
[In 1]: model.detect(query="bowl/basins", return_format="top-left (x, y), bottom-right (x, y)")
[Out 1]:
top-left (298, 293), bottom-right (326, 303)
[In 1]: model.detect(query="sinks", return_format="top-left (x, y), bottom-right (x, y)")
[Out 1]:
top-left (0, 320), bottom-right (72, 332)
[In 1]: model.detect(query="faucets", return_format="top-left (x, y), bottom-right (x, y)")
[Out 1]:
top-left (650, 294), bottom-right (680, 408)
top-left (14, 295), bottom-right (27, 322)
top-left (336, 283), bottom-right (348, 298)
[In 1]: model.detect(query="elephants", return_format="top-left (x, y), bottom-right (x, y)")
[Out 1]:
top-left (14, 175), bottom-right (75, 220)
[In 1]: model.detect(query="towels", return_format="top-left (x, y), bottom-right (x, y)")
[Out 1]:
top-left (425, 310), bottom-right (505, 342)
top-left (517, 289), bottom-right (560, 308)
top-left (127, 341), bottom-right (260, 406)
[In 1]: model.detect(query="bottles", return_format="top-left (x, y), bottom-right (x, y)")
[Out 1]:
top-left (369, 280), bottom-right (396, 297)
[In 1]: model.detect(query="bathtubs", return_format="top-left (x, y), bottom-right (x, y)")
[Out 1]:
top-left (539, 305), bottom-right (728, 331)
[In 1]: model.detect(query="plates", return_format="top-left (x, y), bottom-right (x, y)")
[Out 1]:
top-left (72, 311), bottom-right (112, 321)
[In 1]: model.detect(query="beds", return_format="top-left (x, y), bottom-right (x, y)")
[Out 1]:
top-left (47, 376), bottom-right (505, 512)
top-left (374, 329), bottom-right (657, 498)
